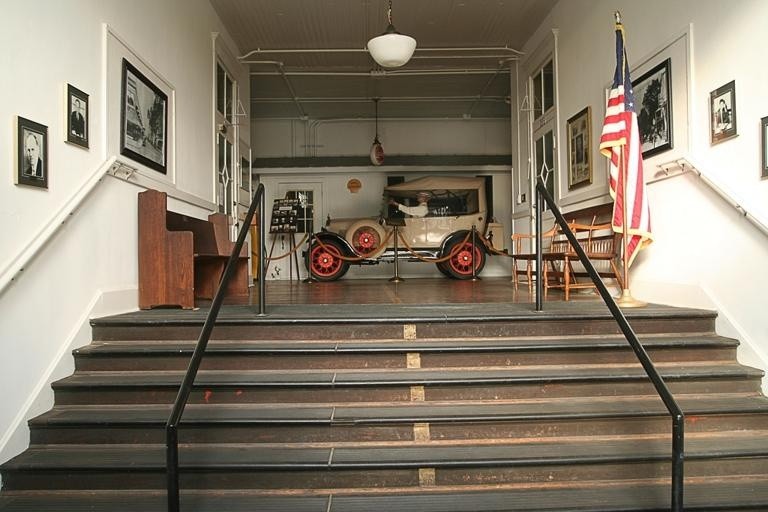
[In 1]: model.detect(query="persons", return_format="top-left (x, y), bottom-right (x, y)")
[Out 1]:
top-left (386, 191), bottom-right (433, 218)
top-left (25, 133), bottom-right (41, 176)
top-left (715, 99), bottom-right (730, 124)
top-left (72, 100), bottom-right (84, 138)
top-left (577, 137), bottom-right (583, 163)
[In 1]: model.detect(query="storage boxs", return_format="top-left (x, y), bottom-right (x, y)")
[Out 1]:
top-left (243, 211), bottom-right (257, 225)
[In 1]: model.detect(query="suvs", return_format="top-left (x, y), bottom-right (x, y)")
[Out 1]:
top-left (300, 175), bottom-right (490, 282)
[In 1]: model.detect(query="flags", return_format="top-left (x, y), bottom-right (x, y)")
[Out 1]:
top-left (598, 22), bottom-right (653, 270)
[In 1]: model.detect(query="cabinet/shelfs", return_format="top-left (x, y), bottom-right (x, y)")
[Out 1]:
top-left (249, 226), bottom-right (260, 280)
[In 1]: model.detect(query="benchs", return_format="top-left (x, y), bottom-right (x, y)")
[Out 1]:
top-left (138, 189), bottom-right (250, 309)
top-left (510, 202), bottom-right (624, 301)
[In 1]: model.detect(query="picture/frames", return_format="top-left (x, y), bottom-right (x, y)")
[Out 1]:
top-left (120, 57), bottom-right (168, 175)
top-left (63, 83), bottom-right (90, 149)
top-left (238, 140), bottom-right (252, 209)
top-left (567, 106), bottom-right (593, 192)
top-left (14, 116), bottom-right (48, 190)
top-left (631, 57), bottom-right (673, 160)
top-left (761, 116), bottom-right (768, 181)
top-left (709, 80), bottom-right (739, 146)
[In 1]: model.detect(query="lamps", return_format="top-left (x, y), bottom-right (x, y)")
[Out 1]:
top-left (367, 0), bottom-right (417, 68)
top-left (370, 98), bottom-right (384, 165)
